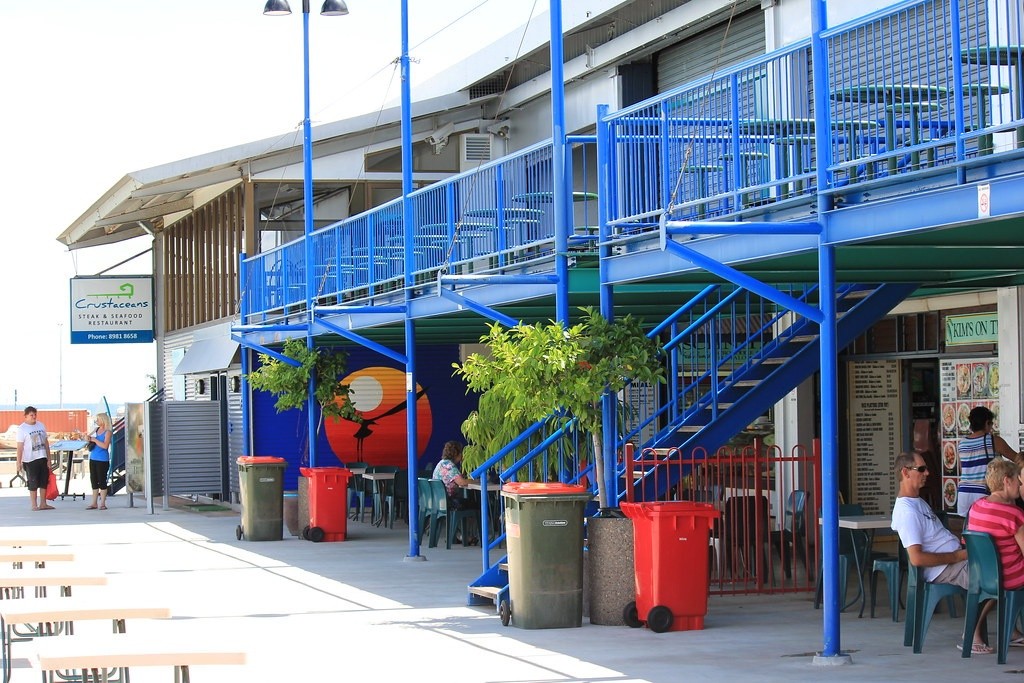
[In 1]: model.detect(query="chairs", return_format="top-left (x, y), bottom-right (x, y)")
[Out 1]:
top-left (682, 485), bottom-right (813, 586)
top-left (70, 449), bottom-right (85, 480)
top-left (347, 474), bottom-right (408, 529)
top-left (813, 506), bottom-right (1024, 664)
top-left (417, 477), bottom-right (482, 549)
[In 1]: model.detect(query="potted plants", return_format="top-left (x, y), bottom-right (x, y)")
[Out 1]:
top-left (449, 307), bottom-right (668, 627)
top-left (240, 339), bottom-right (364, 538)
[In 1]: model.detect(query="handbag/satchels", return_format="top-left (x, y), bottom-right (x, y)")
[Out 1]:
top-left (46, 468), bottom-right (59, 500)
top-left (86, 437), bottom-right (96, 451)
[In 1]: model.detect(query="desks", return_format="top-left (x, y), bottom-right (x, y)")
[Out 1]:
top-left (264, 191), bottom-right (598, 307)
top-left (361, 472), bottom-right (394, 525)
top-left (949, 46), bottom-right (1024, 152)
top-left (459, 478), bottom-right (503, 547)
top-left (819, 513), bottom-right (893, 618)
top-left (50, 438), bottom-right (89, 501)
top-left (349, 467), bottom-right (366, 476)
top-left (30, 634), bottom-right (246, 683)
top-left (0, 530), bottom-right (173, 682)
top-left (828, 85), bottom-right (948, 172)
top-left (725, 119), bottom-right (816, 203)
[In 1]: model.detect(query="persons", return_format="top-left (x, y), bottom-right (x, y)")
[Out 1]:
top-left (85, 413), bottom-right (113, 510)
top-left (957, 406), bottom-right (1018, 519)
top-left (16, 406), bottom-right (55, 511)
top-left (891, 451), bottom-right (1024, 654)
top-left (432, 441), bottom-right (490, 546)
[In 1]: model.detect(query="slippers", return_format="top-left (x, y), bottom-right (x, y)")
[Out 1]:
top-left (99, 506), bottom-right (107, 510)
top-left (957, 642), bottom-right (995, 654)
top-left (31, 505), bottom-right (55, 511)
top-left (1008, 637), bottom-right (1024, 646)
top-left (86, 506), bottom-right (98, 510)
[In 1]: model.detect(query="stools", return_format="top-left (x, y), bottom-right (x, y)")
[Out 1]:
top-left (675, 85), bottom-right (1012, 222)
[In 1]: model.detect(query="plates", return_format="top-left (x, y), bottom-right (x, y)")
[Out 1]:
top-left (942, 363), bottom-right (999, 506)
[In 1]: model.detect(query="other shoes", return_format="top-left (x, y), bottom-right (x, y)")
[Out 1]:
top-left (454, 537), bottom-right (479, 546)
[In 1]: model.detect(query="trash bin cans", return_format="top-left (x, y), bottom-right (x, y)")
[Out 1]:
top-left (619, 500), bottom-right (720, 633)
top-left (236, 456), bottom-right (288, 541)
top-left (300, 467), bottom-right (353, 542)
top-left (501, 482), bottom-right (590, 629)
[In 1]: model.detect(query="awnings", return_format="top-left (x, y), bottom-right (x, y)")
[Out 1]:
top-left (172, 333), bottom-right (242, 374)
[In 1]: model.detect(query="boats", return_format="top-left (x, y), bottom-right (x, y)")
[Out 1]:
top-left (0, 424), bottom-right (20, 449)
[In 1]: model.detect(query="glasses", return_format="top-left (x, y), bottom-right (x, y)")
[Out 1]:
top-left (905, 465), bottom-right (928, 472)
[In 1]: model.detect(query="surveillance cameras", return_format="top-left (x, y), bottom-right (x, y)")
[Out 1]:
top-left (425, 121), bottom-right (455, 145)
